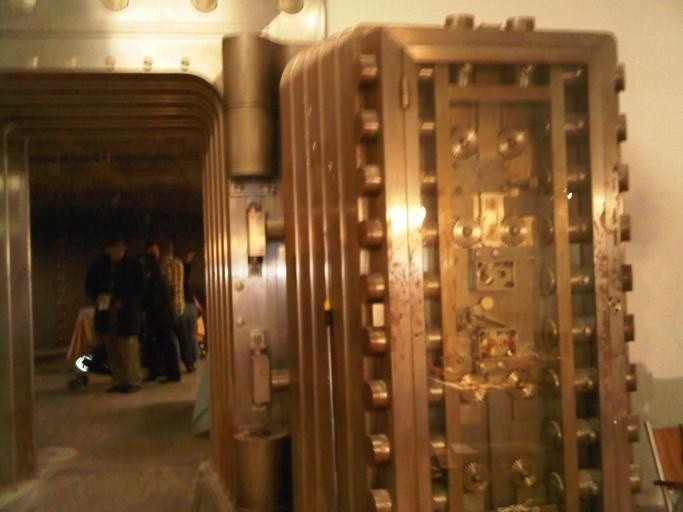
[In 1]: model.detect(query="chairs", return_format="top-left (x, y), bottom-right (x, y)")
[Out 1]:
top-left (643, 416), bottom-right (682, 512)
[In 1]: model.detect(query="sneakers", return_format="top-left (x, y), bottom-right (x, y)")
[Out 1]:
top-left (106, 357), bottom-right (197, 394)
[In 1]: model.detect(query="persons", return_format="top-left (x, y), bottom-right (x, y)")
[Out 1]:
top-left (83, 237), bottom-right (205, 394)
